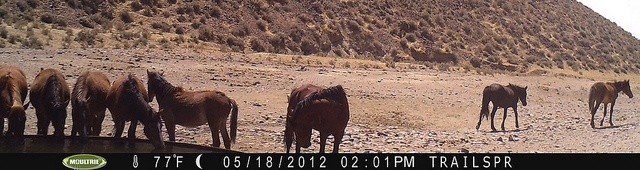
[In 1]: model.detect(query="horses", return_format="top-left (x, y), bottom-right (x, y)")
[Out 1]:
top-left (106, 73), bottom-right (166, 152)
top-left (71, 71), bottom-right (110, 136)
top-left (589, 79), bottom-right (633, 129)
top-left (476, 82), bottom-right (528, 132)
top-left (282, 83), bottom-right (349, 154)
top-left (30, 68), bottom-right (70, 135)
top-left (0, 66), bottom-right (31, 135)
top-left (147, 69), bottom-right (238, 150)
